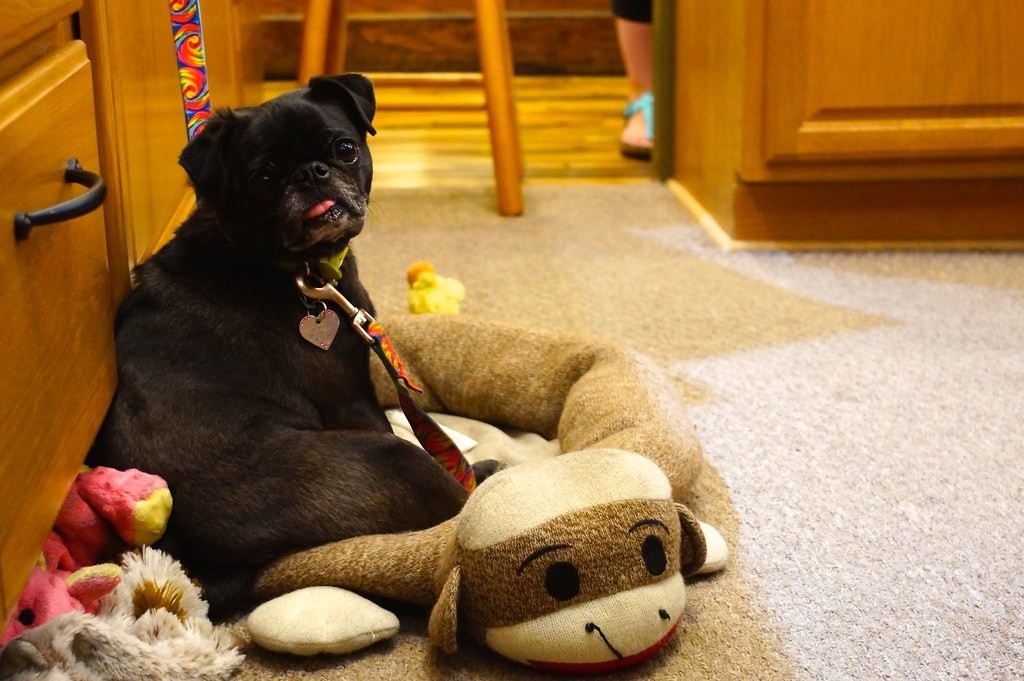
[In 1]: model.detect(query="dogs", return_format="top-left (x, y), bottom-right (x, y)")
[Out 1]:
top-left (92, 74), bottom-right (506, 622)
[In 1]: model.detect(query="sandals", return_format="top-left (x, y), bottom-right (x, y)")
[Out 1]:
top-left (623, 91), bottom-right (654, 157)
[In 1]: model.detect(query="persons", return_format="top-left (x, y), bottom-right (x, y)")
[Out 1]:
top-left (611, 0), bottom-right (659, 154)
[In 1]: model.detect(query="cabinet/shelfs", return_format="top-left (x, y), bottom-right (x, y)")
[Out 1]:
top-left (666, 0), bottom-right (1023, 254)
top-left (0, 0), bottom-right (251, 619)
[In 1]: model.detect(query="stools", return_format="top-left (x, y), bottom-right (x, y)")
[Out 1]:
top-left (294, 2), bottom-right (528, 230)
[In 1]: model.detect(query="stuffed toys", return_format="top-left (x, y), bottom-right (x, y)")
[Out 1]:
top-left (252, 313), bottom-right (728, 667)
top-left (0, 460), bottom-right (173, 645)
